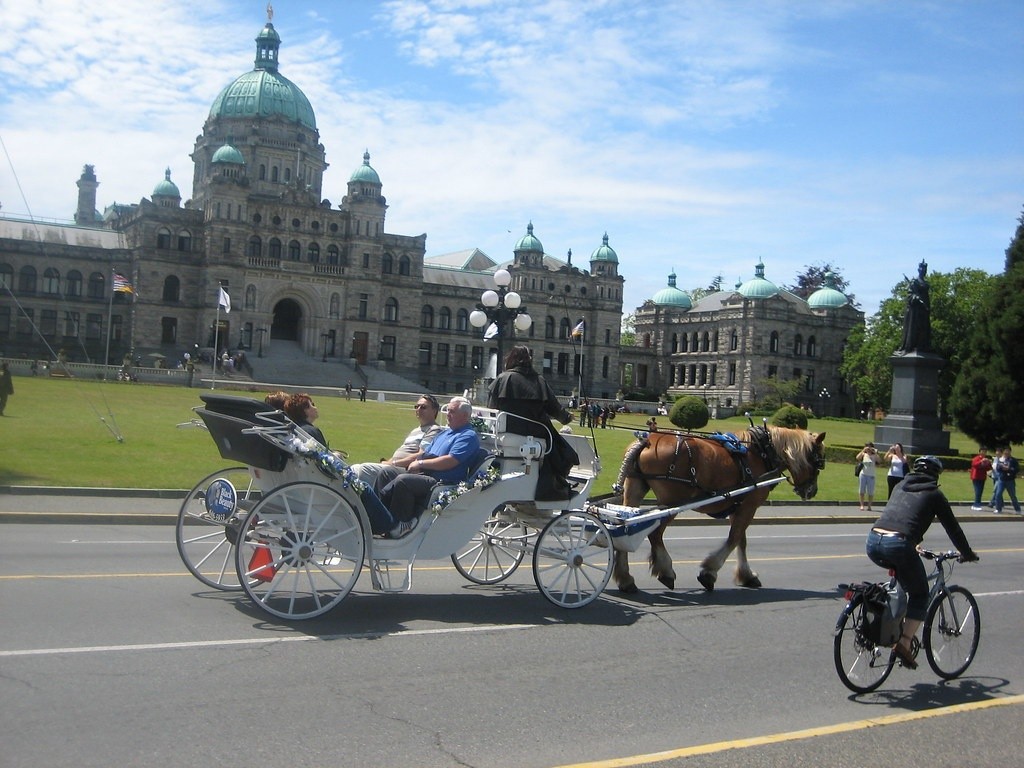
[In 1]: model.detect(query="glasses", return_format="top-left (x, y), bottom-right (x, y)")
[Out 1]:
top-left (415, 405), bottom-right (433, 409)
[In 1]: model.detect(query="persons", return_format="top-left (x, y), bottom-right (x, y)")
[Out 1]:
top-left (154, 359), bottom-right (167, 368)
top-left (177, 352), bottom-right (190, 369)
top-left (30, 360), bottom-right (51, 377)
top-left (579, 401), bottom-right (630, 428)
top-left (266, 380), bottom-right (418, 540)
top-left (209, 345), bottom-right (242, 371)
top-left (491, 347), bottom-right (580, 496)
top-left (971, 447), bottom-right (1022, 515)
top-left (374, 396), bottom-right (480, 521)
top-left (567, 248), bottom-right (572, 264)
top-left (0, 363), bottom-right (14, 415)
top-left (568, 395), bottom-right (577, 409)
top-left (348, 394), bottom-right (443, 487)
top-left (866, 456), bottom-right (979, 670)
top-left (884, 443), bottom-right (909, 499)
top-left (899, 258), bottom-right (933, 352)
top-left (856, 442), bottom-right (882, 511)
top-left (647, 417), bottom-right (657, 431)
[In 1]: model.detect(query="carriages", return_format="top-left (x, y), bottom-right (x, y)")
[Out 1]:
top-left (175, 395), bottom-right (827, 621)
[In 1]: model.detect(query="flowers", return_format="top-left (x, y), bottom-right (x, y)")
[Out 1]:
top-left (430, 466), bottom-right (499, 516)
top-left (289, 435), bottom-right (367, 496)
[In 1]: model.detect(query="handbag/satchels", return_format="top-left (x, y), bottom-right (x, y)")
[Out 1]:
top-left (855, 457), bottom-right (864, 477)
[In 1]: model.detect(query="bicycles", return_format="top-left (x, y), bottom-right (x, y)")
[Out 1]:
top-left (833, 550), bottom-right (980, 694)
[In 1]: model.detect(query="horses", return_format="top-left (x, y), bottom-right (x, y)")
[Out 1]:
top-left (612, 423), bottom-right (828, 594)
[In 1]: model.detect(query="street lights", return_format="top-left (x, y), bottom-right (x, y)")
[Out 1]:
top-left (351, 336), bottom-right (357, 358)
top-left (379, 339), bottom-right (384, 360)
top-left (470, 269), bottom-right (532, 378)
top-left (206, 325), bottom-right (214, 347)
top-left (818, 388), bottom-right (831, 417)
top-left (237, 328), bottom-right (245, 350)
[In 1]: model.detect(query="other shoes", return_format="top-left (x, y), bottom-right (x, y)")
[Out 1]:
top-left (893, 643), bottom-right (918, 670)
top-left (867, 506), bottom-right (871, 511)
top-left (861, 506), bottom-right (864, 510)
top-left (971, 506), bottom-right (982, 510)
top-left (993, 510), bottom-right (1002, 514)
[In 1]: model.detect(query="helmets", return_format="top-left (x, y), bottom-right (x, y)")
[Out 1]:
top-left (914, 455), bottom-right (943, 474)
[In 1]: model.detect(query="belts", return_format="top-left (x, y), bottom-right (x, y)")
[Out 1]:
top-left (874, 531), bottom-right (903, 536)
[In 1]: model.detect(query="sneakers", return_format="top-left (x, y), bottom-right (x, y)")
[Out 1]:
top-left (390, 517), bottom-right (418, 539)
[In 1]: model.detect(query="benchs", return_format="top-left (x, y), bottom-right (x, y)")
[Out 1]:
top-left (427, 447), bottom-right (496, 508)
top-left (440, 403), bottom-right (552, 458)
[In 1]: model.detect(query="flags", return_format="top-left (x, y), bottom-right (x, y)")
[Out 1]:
top-left (112, 274), bottom-right (135, 294)
top-left (482, 323), bottom-right (498, 341)
top-left (220, 288), bottom-right (230, 313)
top-left (573, 322), bottom-right (584, 335)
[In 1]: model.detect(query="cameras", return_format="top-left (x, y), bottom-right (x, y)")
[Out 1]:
top-left (866, 447), bottom-right (872, 452)
top-left (892, 446), bottom-right (897, 451)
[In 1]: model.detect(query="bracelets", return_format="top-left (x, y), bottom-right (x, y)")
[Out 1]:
top-left (393, 460), bottom-right (395, 466)
top-left (419, 461), bottom-right (423, 466)
